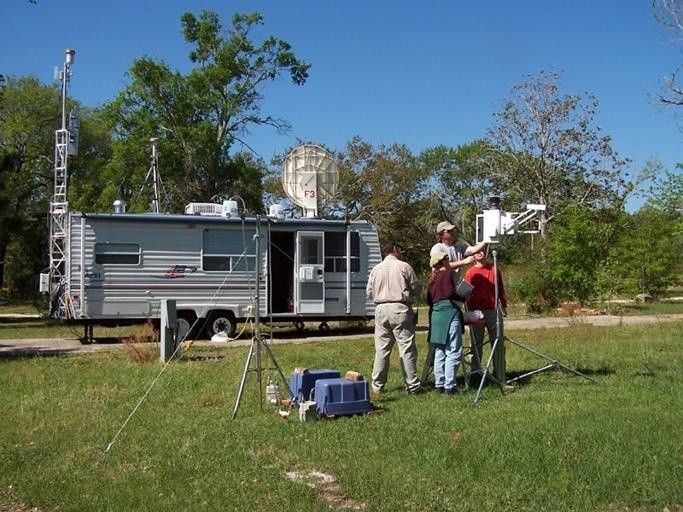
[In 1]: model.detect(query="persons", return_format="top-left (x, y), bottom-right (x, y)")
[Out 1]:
top-left (463, 248), bottom-right (515, 396)
top-left (429, 220), bottom-right (487, 288)
top-left (363, 240), bottom-right (424, 395)
top-left (424, 251), bottom-right (471, 397)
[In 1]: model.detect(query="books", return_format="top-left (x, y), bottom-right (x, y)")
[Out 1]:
top-left (451, 276), bottom-right (474, 310)
top-left (496, 298), bottom-right (507, 317)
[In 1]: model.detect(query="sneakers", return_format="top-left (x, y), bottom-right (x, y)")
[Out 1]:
top-left (408, 387), bottom-right (428, 397)
top-left (437, 387), bottom-right (465, 396)
top-left (372, 382), bottom-right (383, 394)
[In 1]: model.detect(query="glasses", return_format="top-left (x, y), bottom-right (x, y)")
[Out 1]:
top-left (443, 229), bottom-right (456, 233)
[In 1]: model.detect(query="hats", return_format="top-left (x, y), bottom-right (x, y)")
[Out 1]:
top-left (431, 251), bottom-right (447, 267)
top-left (437, 221), bottom-right (456, 233)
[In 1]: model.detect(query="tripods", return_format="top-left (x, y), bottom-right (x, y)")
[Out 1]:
top-left (462, 246), bottom-right (599, 404)
top-left (230, 214), bottom-right (295, 421)
